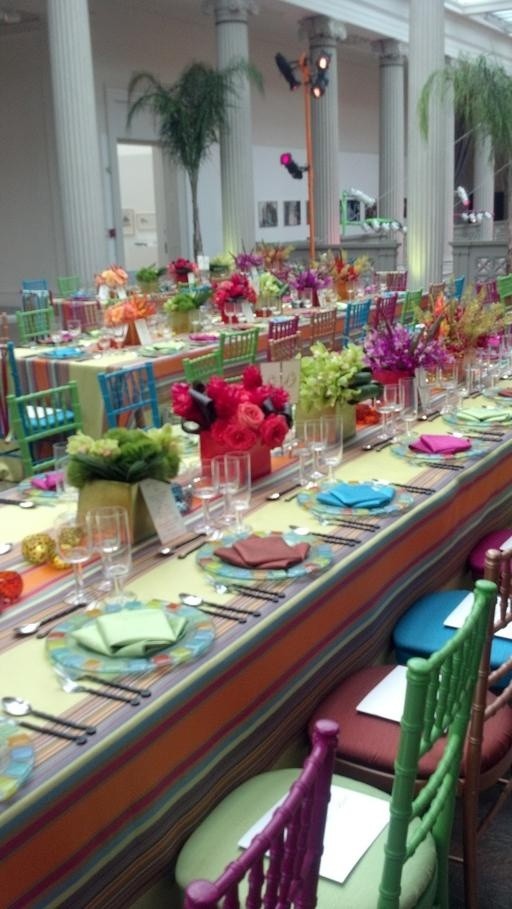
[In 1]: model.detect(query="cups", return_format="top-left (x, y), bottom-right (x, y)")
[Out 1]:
top-left (303, 287), bottom-right (313, 312)
top-left (52, 440), bottom-right (80, 502)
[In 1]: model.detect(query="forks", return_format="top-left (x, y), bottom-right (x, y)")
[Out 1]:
top-left (203, 574), bottom-right (286, 604)
top-left (52, 661), bottom-right (153, 709)
top-left (310, 508), bottom-right (382, 534)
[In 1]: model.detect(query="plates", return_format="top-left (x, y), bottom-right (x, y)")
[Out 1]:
top-left (442, 405), bottom-right (512, 428)
top-left (195, 528), bottom-right (335, 582)
top-left (295, 479), bottom-right (416, 517)
top-left (43, 596), bottom-right (217, 674)
top-left (0, 712), bottom-right (36, 804)
top-left (17, 469), bottom-right (81, 498)
top-left (481, 386), bottom-right (512, 402)
top-left (390, 431), bottom-right (489, 462)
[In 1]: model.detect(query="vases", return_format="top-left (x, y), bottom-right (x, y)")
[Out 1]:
top-left (299, 397), bottom-right (357, 439)
top-left (375, 369), bottom-right (414, 408)
top-left (199, 430), bottom-right (272, 483)
top-left (75, 479), bottom-right (167, 542)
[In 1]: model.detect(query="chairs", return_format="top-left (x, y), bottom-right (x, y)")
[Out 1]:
top-left (469, 522), bottom-right (512, 579)
top-left (5, 265), bottom-right (512, 355)
top-left (1, 340), bottom-right (77, 478)
top-left (98, 361), bottom-right (162, 433)
top-left (176, 578), bottom-right (501, 907)
top-left (305, 545), bottom-right (512, 906)
top-left (184, 718), bottom-right (340, 908)
top-left (264, 312), bottom-right (301, 362)
top-left (5, 379), bottom-right (85, 480)
top-left (393, 586), bottom-right (511, 696)
top-left (220, 326), bottom-right (261, 383)
top-left (182, 349), bottom-right (223, 385)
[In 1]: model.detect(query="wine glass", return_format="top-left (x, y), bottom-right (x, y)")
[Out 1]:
top-left (188, 450), bottom-right (252, 543)
top-left (370, 376), bottom-right (420, 446)
top-left (53, 504), bottom-right (138, 605)
top-left (266, 292), bottom-right (283, 325)
top-left (48, 321), bottom-right (64, 357)
top-left (487, 363), bottom-right (502, 391)
top-left (288, 413), bottom-right (344, 491)
top-left (66, 319), bottom-right (83, 350)
top-left (97, 322), bottom-right (127, 362)
top-left (222, 302), bottom-right (238, 331)
top-left (187, 309), bottom-right (202, 338)
top-left (344, 279), bottom-right (356, 304)
top-left (437, 360), bottom-right (472, 416)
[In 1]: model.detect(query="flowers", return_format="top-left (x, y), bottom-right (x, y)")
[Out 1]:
top-left (167, 361), bottom-right (295, 452)
top-left (62, 423), bottom-right (183, 486)
top-left (292, 338), bottom-right (384, 411)
top-left (360, 323), bottom-right (457, 369)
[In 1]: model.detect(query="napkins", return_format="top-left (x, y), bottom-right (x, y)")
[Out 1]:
top-left (456, 408), bottom-right (512, 424)
top-left (497, 387), bottom-right (512, 398)
top-left (29, 473), bottom-right (67, 492)
top-left (408, 434), bottom-right (471, 456)
top-left (214, 533), bottom-right (310, 571)
top-left (316, 482), bottom-right (397, 509)
top-left (72, 609), bottom-right (190, 658)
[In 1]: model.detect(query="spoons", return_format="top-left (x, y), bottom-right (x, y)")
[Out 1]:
top-left (263, 483), bottom-right (305, 502)
top-left (416, 410), bottom-right (442, 423)
top-left (1, 695), bottom-right (98, 737)
top-left (176, 590), bottom-right (263, 624)
top-left (154, 532), bottom-right (211, 557)
top-left (12, 602), bottom-right (93, 639)
top-left (360, 436), bottom-right (395, 453)
top-left (289, 523), bottom-right (363, 549)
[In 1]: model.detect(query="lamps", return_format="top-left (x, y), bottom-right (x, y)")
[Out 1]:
top-left (351, 188), bottom-right (374, 207)
top-left (306, 50), bottom-right (332, 98)
top-left (461, 208), bottom-right (493, 223)
top-left (454, 186), bottom-right (469, 205)
top-left (275, 51), bottom-right (301, 93)
top-left (281, 153), bottom-right (308, 180)
top-left (362, 219), bottom-right (407, 235)
